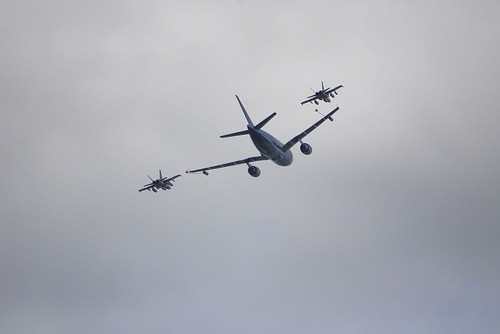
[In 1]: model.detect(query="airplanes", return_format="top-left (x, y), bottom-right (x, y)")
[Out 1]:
top-left (185, 94), bottom-right (340, 177)
top-left (301, 80), bottom-right (344, 105)
top-left (138, 169), bottom-right (182, 193)
